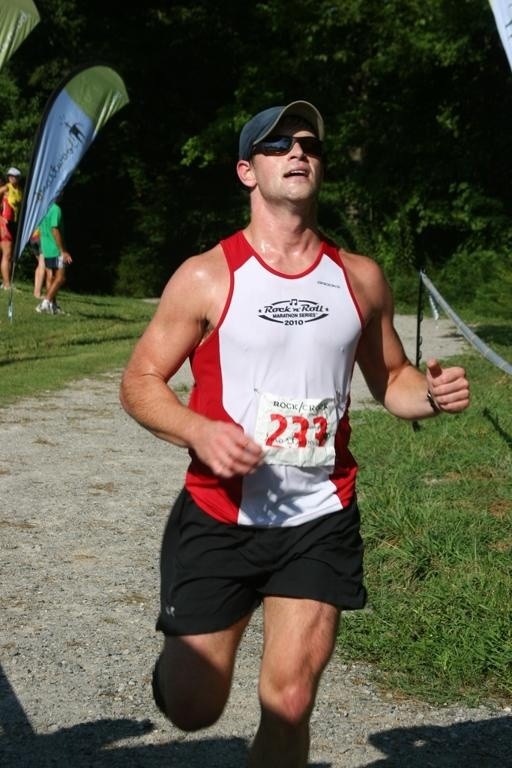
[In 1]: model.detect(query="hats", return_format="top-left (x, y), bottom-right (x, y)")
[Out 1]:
top-left (238, 100), bottom-right (325, 161)
top-left (6, 167), bottom-right (21, 176)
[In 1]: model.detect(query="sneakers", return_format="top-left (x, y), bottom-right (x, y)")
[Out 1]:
top-left (36, 303), bottom-right (64, 315)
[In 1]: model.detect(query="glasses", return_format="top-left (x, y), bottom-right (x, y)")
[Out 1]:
top-left (254, 134), bottom-right (323, 156)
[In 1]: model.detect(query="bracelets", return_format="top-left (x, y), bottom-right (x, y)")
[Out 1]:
top-left (425, 386), bottom-right (443, 413)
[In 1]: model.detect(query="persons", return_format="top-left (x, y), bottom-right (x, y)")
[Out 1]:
top-left (0, 165), bottom-right (24, 293)
top-left (31, 186), bottom-right (74, 316)
top-left (119, 101), bottom-right (476, 766)
top-left (30, 224), bottom-right (55, 301)
